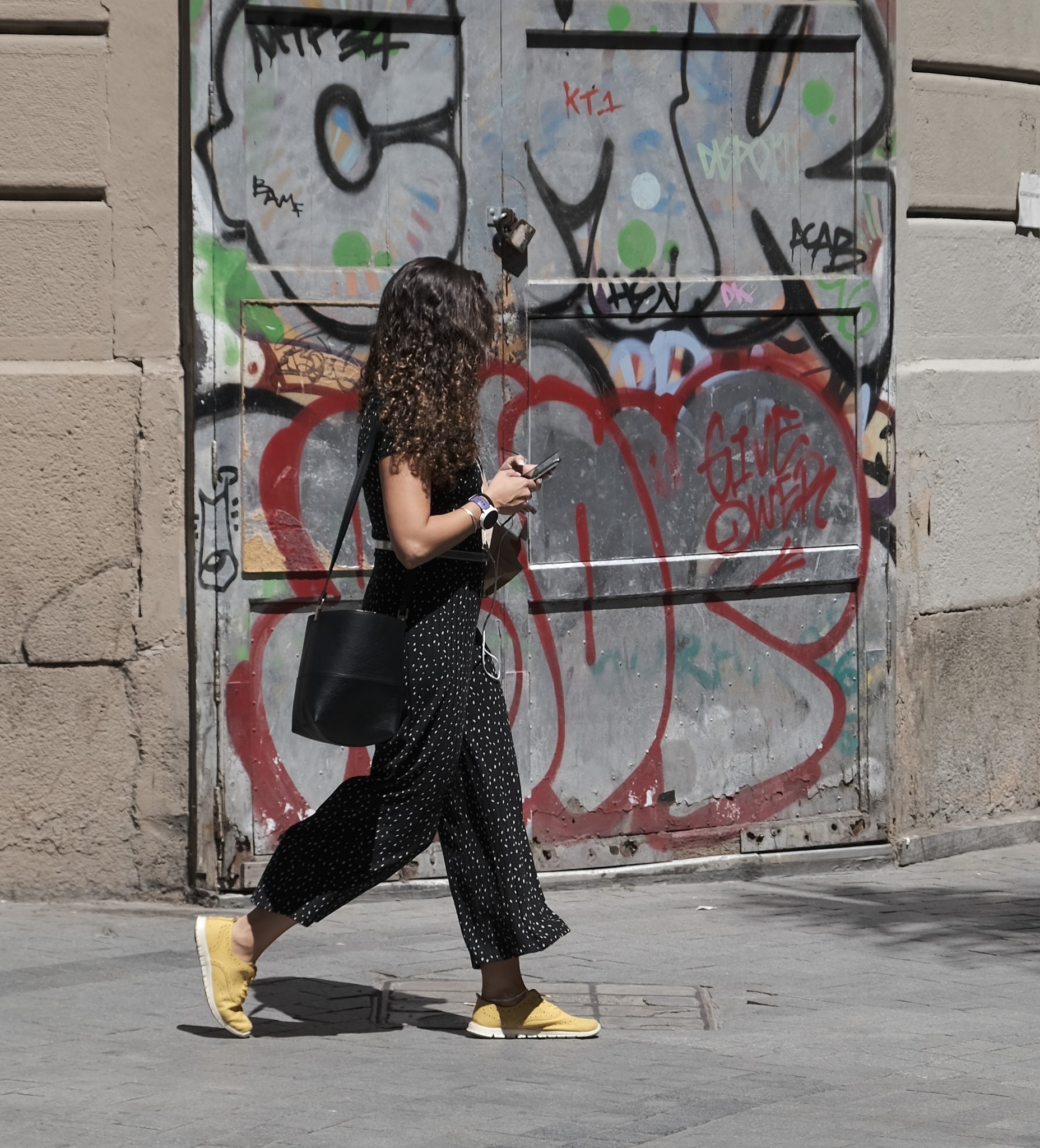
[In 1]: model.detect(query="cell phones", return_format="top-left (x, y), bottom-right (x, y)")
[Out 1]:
top-left (522, 450), bottom-right (562, 480)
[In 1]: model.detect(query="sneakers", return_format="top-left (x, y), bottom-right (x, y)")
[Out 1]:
top-left (465, 988), bottom-right (602, 1039)
top-left (194, 915), bottom-right (257, 1037)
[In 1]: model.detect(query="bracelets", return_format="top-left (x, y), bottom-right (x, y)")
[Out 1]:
top-left (479, 493), bottom-right (495, 508)
top-left (459, 508), bottom-right (477, 534)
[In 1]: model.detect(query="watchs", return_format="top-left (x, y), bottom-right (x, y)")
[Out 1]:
top-left (468, 494), bottom-right (499, 531)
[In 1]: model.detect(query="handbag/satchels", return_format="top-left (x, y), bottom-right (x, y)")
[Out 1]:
top-left (290, 609), bottom-right (407, 745)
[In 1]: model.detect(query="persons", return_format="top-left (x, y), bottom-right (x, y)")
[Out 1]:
top-left (194, 256), bottom-right (599, 1042)
